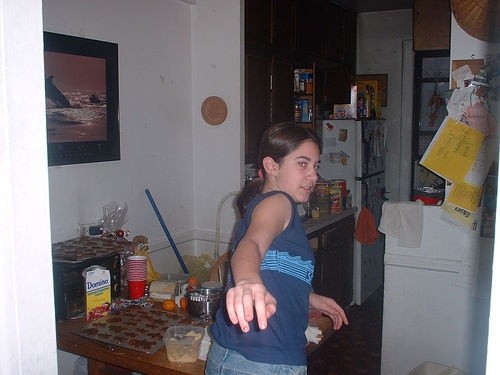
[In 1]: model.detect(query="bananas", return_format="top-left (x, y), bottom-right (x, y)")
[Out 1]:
top-left (135, 246), bottom-right (156, 282)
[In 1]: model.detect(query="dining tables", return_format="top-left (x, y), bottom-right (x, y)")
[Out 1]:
top-left (55, 289), bottom-right (343, 375)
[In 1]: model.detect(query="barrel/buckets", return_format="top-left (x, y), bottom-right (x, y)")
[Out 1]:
top-left (316, 182), bottom-right (330, 213)
top-left (329, 179), bottom-right (346, 213)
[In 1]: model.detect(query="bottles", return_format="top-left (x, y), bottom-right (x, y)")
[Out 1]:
top-left (133, 235), bottom-right (158, 296)
top-left (305, 201), bottom-right (312, 220)
top-left (294, 71), bottom-right (313, 95)
top-left (357, 86), bottom-right (376, 118)
top-left (118, 251), bottom-right (133, 292)
top-left (294, 98), bottom-right (313, 124)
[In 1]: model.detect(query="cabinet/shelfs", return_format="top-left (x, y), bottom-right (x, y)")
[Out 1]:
top-left (303, 209), bottom-right (354, 305)
top-left (412, 1), bottom-right (450, 51)
top-left (245, 1), bottom-right (339, 125)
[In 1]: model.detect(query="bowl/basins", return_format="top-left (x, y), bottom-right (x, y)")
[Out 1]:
top-left (413, 187), bottom-right (442, 204)
top-left (162, 325), bottom-right (205, 363)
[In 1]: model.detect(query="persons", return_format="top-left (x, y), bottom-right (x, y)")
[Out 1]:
top-left (205, 122), bottom-right (348, 375)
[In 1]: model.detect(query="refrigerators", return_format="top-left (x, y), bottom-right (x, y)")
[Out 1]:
top-left (321, 118), bottom-right (388, 306)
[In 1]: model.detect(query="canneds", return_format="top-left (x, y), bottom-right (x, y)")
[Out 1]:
top-left (314, 179), bottom-right (347, 214)
top-left (294, 71), bottom-right (313, 122)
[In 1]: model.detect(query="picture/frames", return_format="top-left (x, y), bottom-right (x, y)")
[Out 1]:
top-left (43, 30), bottom-right (123, 166)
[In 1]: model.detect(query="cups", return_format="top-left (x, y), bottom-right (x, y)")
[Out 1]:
top-left (126, 256), bottom-right (147, 301)
top-left (311, 210), bottom-right (320, 218)
top-left (184, 288), bottom-right (225, 322)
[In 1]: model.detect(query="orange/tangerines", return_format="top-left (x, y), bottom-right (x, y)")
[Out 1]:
top-left (163, 278), bottom-right (198, 311)
